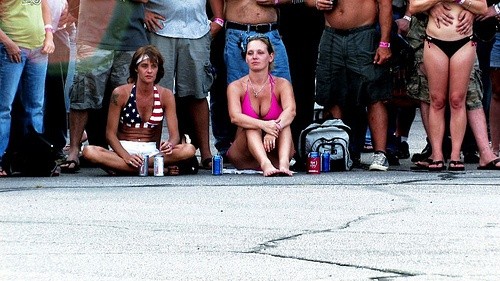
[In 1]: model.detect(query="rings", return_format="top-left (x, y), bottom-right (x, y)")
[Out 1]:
top-left (129, 162), bottom-right (133, 166)
top-left (269, 141), bottom-right (272, 144)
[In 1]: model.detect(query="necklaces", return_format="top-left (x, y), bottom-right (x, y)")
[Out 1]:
top-left (248, 75), bottom-right (269, 97)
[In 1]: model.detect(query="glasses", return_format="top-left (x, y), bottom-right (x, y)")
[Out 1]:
top-left (246, 35), bottom-right (271, 46)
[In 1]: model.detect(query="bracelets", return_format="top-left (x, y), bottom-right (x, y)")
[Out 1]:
top-left (213, 18), bottom-right (224, 26)
top-left (465, 0), bottom-right (471, 9)
top-left (494, 4), bottom-right (500, 14)
top-left (379, 42), bottom-right (391, 48)
top-left (403, 15), bottom-right (412, 22)
top-left (44, 24), bottom-right (53, 31)
top-left (457, 0), bottom-right (465, 5)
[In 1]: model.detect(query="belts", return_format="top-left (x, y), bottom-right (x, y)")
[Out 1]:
top-left (227, 21), bottom-right (278, 33)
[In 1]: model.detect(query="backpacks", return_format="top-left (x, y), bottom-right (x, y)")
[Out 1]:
top-left (297, 109), bottom-right (353, 171)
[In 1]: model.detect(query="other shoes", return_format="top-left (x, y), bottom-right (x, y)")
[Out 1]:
top-left (398, 141), bottom-right (410, 158)
top-left (411, 137), bottom-right (453, 162)
top-left (464, 151), bottom-right (481, 163)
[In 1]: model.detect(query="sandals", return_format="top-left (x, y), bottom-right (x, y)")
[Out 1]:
top-left (0, 169), bottom-right (8, 177)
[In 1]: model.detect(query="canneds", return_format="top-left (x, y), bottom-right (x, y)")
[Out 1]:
top-left (308, 152), bottom-right (319, 158)
top-left (320, 153), bottom-right (331, 172)
top-left (154, 155), bottom-right (164, 176)
top-left (138, 155), bottom-right (149, 177)
top-left (212, 155), bottom-right (223, 175)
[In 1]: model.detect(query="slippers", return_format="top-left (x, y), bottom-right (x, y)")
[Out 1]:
top-left (429, 161), bottom-right (446, 170)
top-left (64, 138), bottom-right (88, 151)
top-left (477, 159), bottom-right (500, 169)
top-left (62, 159), bottom-right (80, 173)
top-left (410, 159), bottom-right (432, 170)
top-left (448, 161), bottom-right (465, 171)
top-left (198, 157), bottom-right (225, 169)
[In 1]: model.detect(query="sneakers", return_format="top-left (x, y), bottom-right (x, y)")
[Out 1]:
top-left (369, 153), bottom-right (389, 170)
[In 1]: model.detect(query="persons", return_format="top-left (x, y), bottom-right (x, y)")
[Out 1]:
top-left (82, 44), bottom-right (196, 177)
top-left (0, 0), bottom-right (500, 171)
top-left (226, 36), bottom-right (297, 177)
